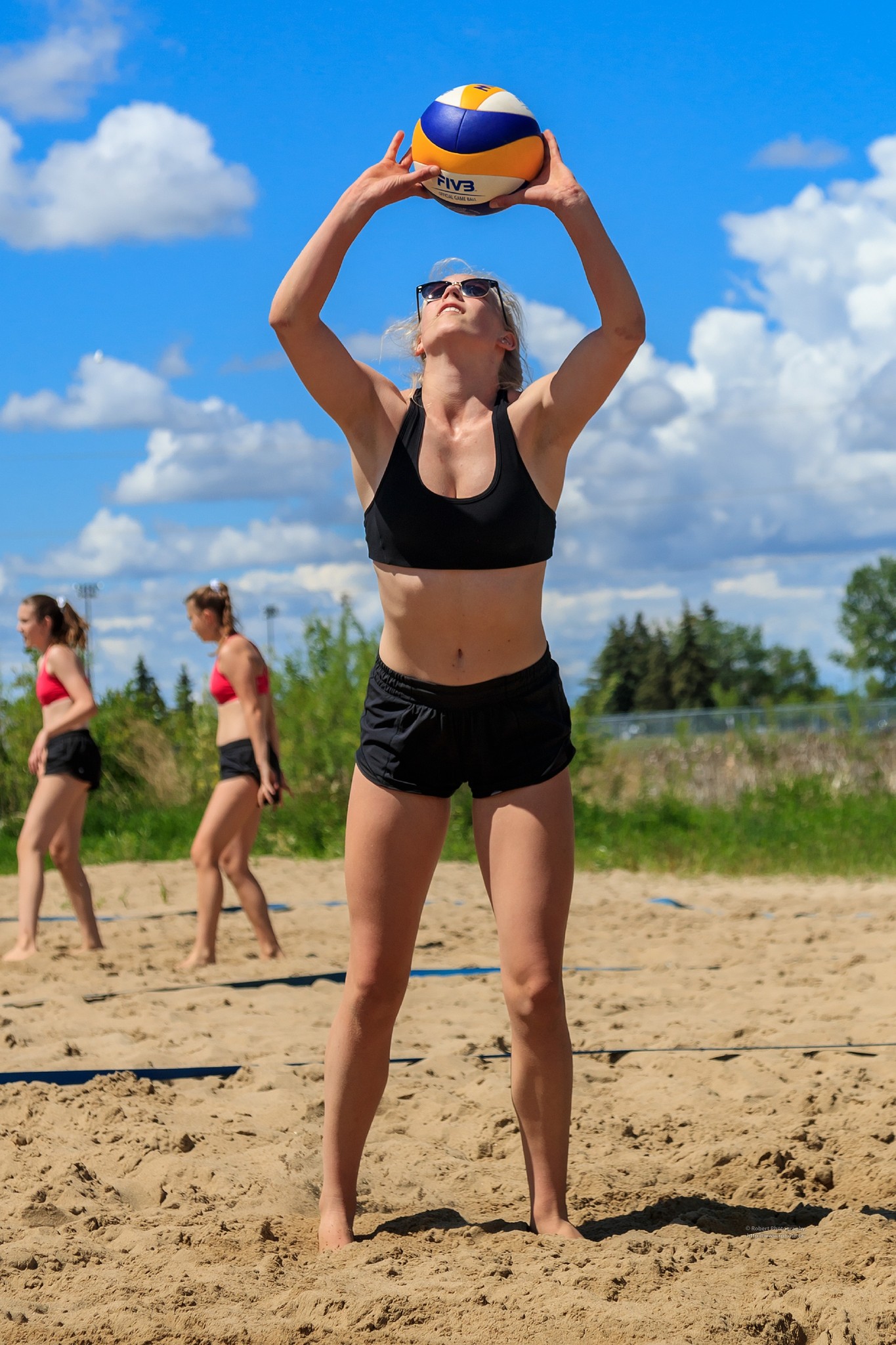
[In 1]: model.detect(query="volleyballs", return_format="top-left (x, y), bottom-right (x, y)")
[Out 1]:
top-left (410, 82), bottom-right (544, 216)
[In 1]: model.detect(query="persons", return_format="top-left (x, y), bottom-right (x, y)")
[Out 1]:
top-left (268, 130), bottom-right (646, 1253)
top-left (2, 595), bottom-right (104, 960)
top-left (183, 579), bottom-right (294, 968)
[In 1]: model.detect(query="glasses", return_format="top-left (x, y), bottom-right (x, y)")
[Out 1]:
top-left (417, 278), bottom-right (508, 326)
